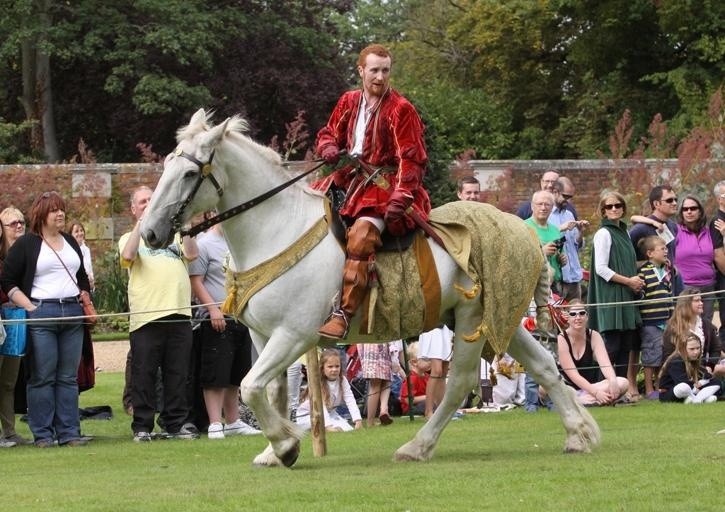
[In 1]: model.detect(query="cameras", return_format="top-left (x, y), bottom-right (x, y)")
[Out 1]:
top-left (553, 236), bottom-right (566, 250)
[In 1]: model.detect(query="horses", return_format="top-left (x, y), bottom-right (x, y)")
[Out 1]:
top-left (138, 107), bottom-right (602, 468)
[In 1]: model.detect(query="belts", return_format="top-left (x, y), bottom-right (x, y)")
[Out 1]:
top-left (30, 297), bottom-right (79, 303)
top-left (533, 335), bottom-right (557, 342)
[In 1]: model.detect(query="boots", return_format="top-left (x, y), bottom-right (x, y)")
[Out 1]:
top-left (317, 220), bottom-right (383, 341)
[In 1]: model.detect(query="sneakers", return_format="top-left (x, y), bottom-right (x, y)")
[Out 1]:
top-left (132, 420), bottom-right (264, 442)
top-left (379, 412), bottom-right (393, 425)
top-left (5, 435), bottom-right (94, 447)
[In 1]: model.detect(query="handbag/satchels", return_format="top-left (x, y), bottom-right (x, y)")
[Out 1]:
top-left (81, 290), bottom-right (98, 324)
top-left (0, 306), bottom-right (28, 357)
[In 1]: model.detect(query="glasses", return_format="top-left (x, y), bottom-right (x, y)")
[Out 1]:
top-left (566, 311), bottom-right (588, 318)
top-left (36, 191), bottom-right (63, 206)
top-left (4, 221), bottom-right (27, 228)
top-left (563, 195), bottom-right (698, 253)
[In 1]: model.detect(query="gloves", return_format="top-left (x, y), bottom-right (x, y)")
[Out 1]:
top-left (383, 190), bottom-right (416, 238)
top-left (316, 139), bottom-right (342, 167)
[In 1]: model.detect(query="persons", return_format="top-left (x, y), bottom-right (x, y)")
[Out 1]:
top-left (119, 172), bottom-right (725, 440)
top-left (304, 44), bottom-right (430, 339)
top-left (0, 192), bottom-right (100, 448)
